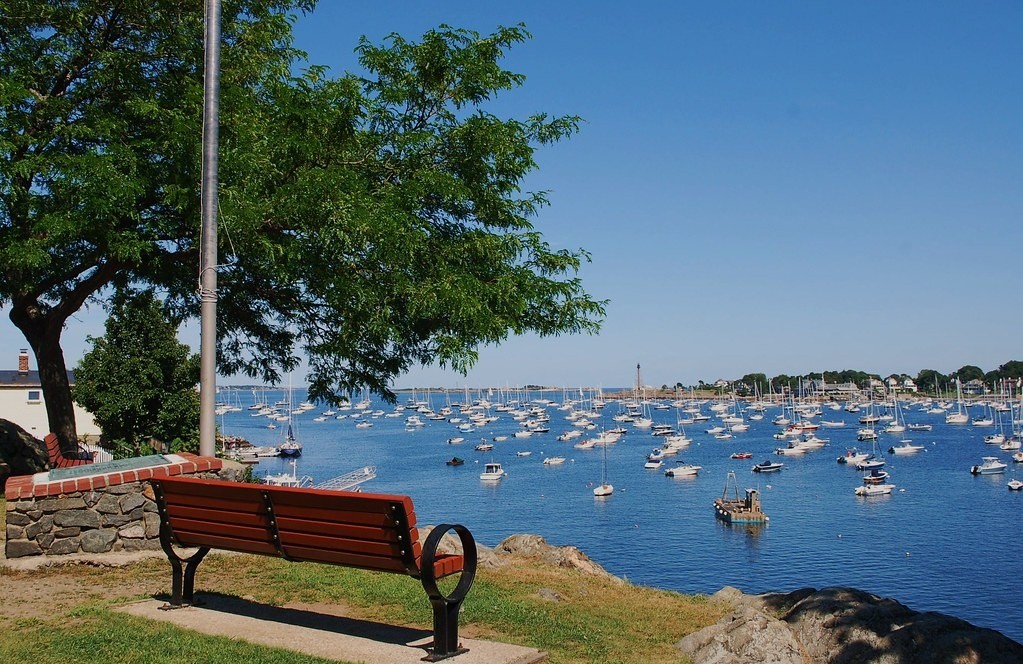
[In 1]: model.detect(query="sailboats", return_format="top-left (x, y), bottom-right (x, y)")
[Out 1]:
top-left (857, 391), bottom-right (878, 441)
top-left (1011, 419), bottom-right (1023, 462)
top-left (594, 420), bottom-right (613, 495)
top-left (215, 378), bottom-right (1023, 440)
top-left (855, 375), bottom-right (887, 471)
top-left (787, 394), bottom-right (801, 448)
top-left (985, 407), bottom-right (1006, 444)
top-left (275, 371), bottom-right (302, 458)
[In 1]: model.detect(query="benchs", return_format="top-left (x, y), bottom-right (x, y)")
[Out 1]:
top-left (43, 431), bottom-right (95, 469)
top-left (146, 473), bottom-right (478, 662)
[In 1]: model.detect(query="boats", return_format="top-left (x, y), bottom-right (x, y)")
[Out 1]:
top-left (646, 439), bottom-right (692, 458)
top-left (644, 459), bottom-right (665, 469)
top-left (445, 457), bottom-right (465, 465)
top-left (557, 430), bottom-right (584, 440)
top-left (652, 430), bottom-right (676, 436)
top-left (480, 456), bottom-right (503, 480)
top-left (999, 440), bottom-right (1022, 450)
top-left (1006, 480), bottom-right (1023, 489)
top-left (511, 430), bottom-right (534, 438)
top-left (664, 460), bottom-right (701, 476)
top-left (836, 446), bottom-right (869, 464)
top-left (970, 455), bottom-right (1007, 474)
top-left (729, 451), bottom-right (752, 459)
top-left (798, 437), bottom-right (829, 450)
top-left (447, 437), bottom-right (464, 443)
top-left (887, 433), bottom-right (924, 454)
top-left (855, 484), bottom-right (895, 495)
top-left (475, 437), bottom-right (494, 451)
top-left (751, 460), bottom-right (783, 473)
top-left (773, 448), bottom-right (806, 456)
top-left (518, 452), bottom-right (532, 456)
top-left (712, 470), bottom-right (770, 523)
top-left (863, 465), bottom-right (888, 484)
top-left (574, 433), bottom-right (621, 449)
top-left (543, 457), bottom-right (566, 464)
top-left (493, 436), bottom-right (508, 441)
top-left (261, 459), bottom-right (313, 488)
